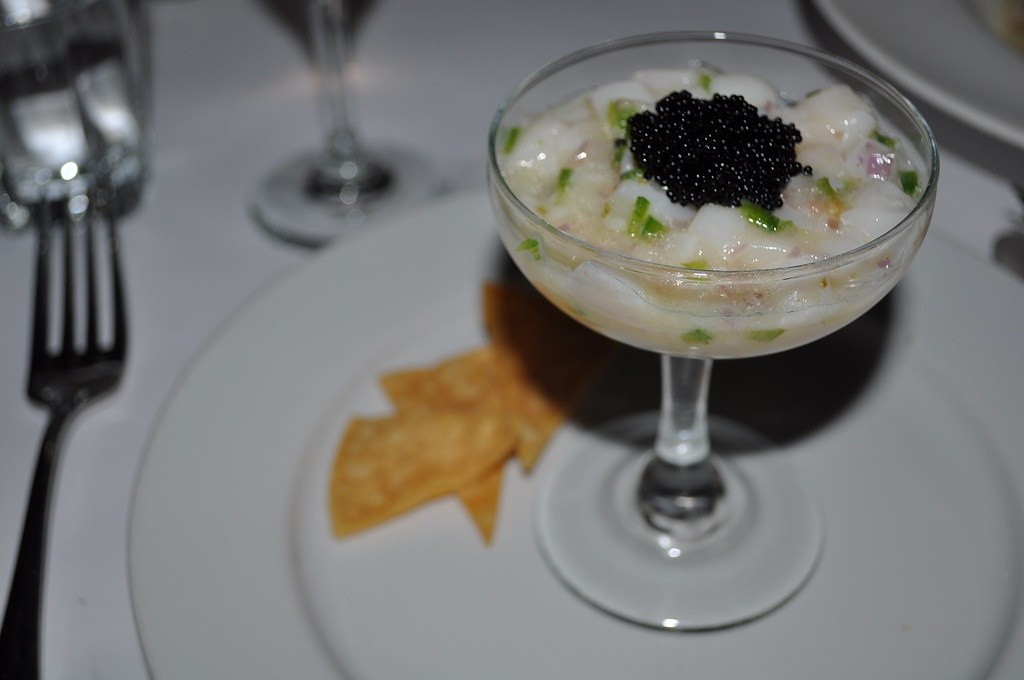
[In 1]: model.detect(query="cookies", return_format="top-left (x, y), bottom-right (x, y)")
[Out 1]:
top-left (330, 282), bottom-right (602, 545)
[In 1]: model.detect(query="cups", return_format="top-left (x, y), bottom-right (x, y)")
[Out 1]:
top-left (0, 0), bottom-right (153, 226)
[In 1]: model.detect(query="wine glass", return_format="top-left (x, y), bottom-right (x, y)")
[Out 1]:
top-left (484, 30), bottom-right (939, 633)
top-left (0, 192), bottom-right (128, 680)
top-left (252, 0), bottom-right (442, 251)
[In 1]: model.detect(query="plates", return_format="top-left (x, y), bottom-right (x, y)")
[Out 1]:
top-left (124, 118), bottom-right (1024, 680)
top-left (802, 0), bottom-right (1023, 158)
